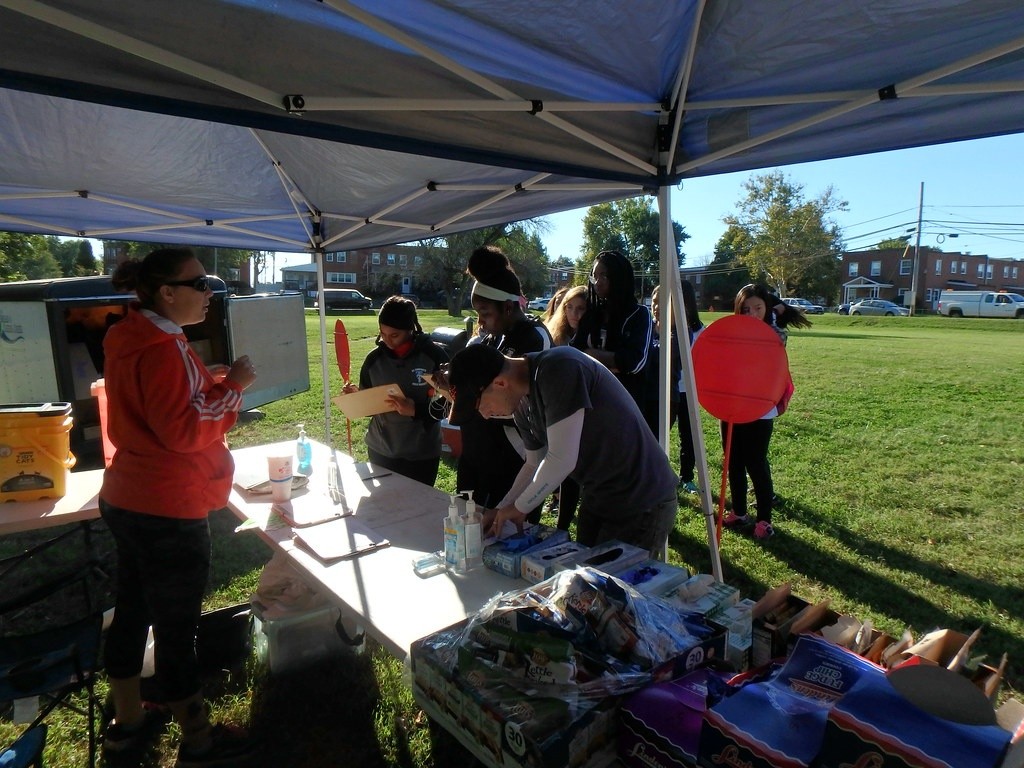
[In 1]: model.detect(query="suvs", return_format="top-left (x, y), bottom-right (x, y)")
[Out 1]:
top-left (781, 298), bottom-right (824, 314)
top-left (838, 297), bottom-right (878, 315)
top-left (400, 294), bottom-right (420, 307)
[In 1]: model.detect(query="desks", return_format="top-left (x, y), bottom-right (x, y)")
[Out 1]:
top-left (227, 437), bottom-right (549, 659)
top-left (0, 468), bottom-right (148, 642)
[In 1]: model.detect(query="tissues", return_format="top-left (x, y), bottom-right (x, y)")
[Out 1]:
top-left (660, 571), bottom-right (741, 620)
top-left (705, 597), bottom-right (760, 671)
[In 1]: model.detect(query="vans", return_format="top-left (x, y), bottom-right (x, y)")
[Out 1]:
top-left (313, 289), bottom-right (373, 313)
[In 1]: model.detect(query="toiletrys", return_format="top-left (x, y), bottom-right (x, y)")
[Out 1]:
top-left (295, 424), bottom-right (312, 466)
top-left (412, 488), bottom-right (484, 575)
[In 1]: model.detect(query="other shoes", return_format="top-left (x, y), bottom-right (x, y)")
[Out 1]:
top-left (106, 707), bottom-right (165, 752)
top-left (175, 724), bottom-right (265, 767)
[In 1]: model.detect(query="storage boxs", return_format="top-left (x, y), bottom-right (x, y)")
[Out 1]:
top-left (414, 524), bottom-right (1024, 768)
top-left (246, 594), bottom-right (366, 677)
top-left (188, 337), bottom-right (214, 365)
top-left (79, 424), bottom-right (100, 439)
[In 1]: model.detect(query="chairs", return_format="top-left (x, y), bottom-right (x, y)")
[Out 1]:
top-left (0, 520), bottom-right (104, 767)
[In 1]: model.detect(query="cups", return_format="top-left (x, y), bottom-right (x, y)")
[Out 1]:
top-left (270, 476), bottom-right (293, 501)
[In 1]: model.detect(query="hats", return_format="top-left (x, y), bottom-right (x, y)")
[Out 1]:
top-left (447, 345), bottom-right (506, 426)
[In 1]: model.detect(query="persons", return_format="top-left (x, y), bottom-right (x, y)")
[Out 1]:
top-left (342, 296), bottom-right (453, 486)
top-left (431, 247), bottom-right (813, 562)
top-left (97, 246), bottom-right (263, 768)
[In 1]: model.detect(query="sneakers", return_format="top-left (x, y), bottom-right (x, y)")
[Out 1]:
top-left (723, 511), bottom-right (750, 526)
top-left (680, 478), bottom-right (699, 495)
top-left (754, 519), bottom-right (774, 540)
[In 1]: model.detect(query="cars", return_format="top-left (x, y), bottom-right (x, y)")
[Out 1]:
top-left (848, 300), bottom-right (908, 316)
top-left (528, 298), bottom-right (549, 311)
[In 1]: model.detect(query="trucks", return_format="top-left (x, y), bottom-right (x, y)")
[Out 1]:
top-left (938, 291), bottom-right (1024, 319)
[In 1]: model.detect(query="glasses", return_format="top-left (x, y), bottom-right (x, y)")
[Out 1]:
top-left (158, 277), bottom-right (210, 292)
top-left (589, 271), bottom-right (609, 286)
top-left (565, 304), bottom-right (586, 314)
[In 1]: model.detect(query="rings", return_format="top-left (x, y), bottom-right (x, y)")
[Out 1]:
top-left (493, 521), bottom-right (497, 524)
